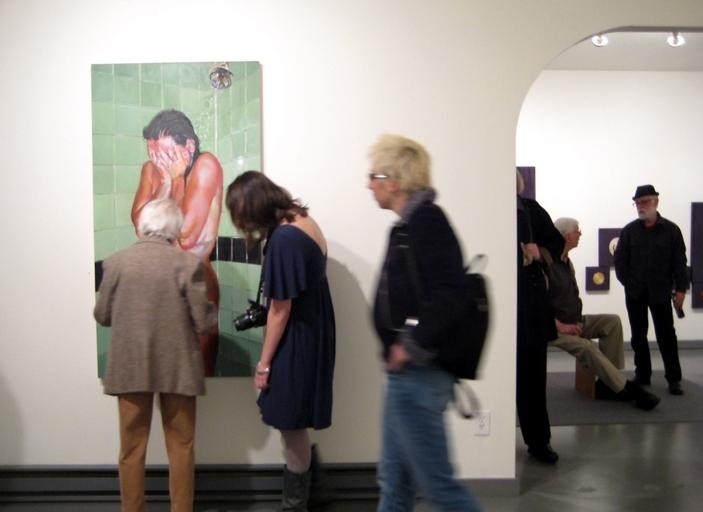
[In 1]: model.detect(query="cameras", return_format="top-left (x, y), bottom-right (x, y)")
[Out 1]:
top-left (233, 296), bottom-right (271, 334)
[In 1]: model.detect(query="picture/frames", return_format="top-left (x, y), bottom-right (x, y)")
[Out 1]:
top-left (90, 60), bottom-right (268, 380)
top-left (597, 226), bottom-right (626, 269)
top-left (585, 265), bottom-right (611, 292)
top-left (516, 166), bottom-right (536, 199)
top-left (690, 201), bottom-right (703, 311)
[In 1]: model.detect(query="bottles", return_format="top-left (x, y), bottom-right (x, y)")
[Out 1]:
top-left (671, 288), bottom-right (685, 319)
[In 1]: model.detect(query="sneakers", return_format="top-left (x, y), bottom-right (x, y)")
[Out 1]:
top-left (634, 376), bottom-right (652, 386)
top-left (528, 446), bottom-right (558, 465)
top-left (669, 382), bottom-right (682, 397)
top-left (596, 379), bottom-right (623, 401)
top-left (620, 381), bottom-right (658, 412)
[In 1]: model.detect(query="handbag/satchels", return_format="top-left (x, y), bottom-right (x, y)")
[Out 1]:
top-left (433, 256), bottom-right (489, 418)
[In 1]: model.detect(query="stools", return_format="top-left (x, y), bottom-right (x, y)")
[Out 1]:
top-left (546, 340), bottom-right (602, 402)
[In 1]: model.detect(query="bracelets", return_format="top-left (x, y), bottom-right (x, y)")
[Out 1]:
top-left (255, 362), bottom-right (270, 376)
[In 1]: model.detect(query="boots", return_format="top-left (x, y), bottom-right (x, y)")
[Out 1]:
top-left (309, 456), bottom-right (326, 512)
top-left (281, 464), bottom-right (310, 512)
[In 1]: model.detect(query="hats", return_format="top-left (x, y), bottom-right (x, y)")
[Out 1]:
top-left (632, 185), bottom-right (659, 199)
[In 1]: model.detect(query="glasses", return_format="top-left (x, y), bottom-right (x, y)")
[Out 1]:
top-left (572, 230), bottom-right (582, 234)
top-left (632, 198), bottom-right (655, 207)
top-left (369, 172), bottom-right (389, 180)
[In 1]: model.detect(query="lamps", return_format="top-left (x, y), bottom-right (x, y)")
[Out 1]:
top-left (665, 31), bottom-right (687, 49)
top-left (590, 33), bottom-right (610, 48)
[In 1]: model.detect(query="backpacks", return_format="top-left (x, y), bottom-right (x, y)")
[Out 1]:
top-left (410, 213), bottom-right (489, 416)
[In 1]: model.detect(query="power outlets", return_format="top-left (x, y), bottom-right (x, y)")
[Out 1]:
top-left (471, 409), bottom-right (492, 438)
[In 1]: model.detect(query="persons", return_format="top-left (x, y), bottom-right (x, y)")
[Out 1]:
top-left (367, 133), bottom-right (483, 512)
top-left (130, 109), bottom-right (220, 377)
top-left (547, 217), bottom-right (661, 413)
top-left (516, 169), bottom-right (566, 465)
top-left (93, 197), bottom-right (217, 512)
top-left (225, 170), bottom-right (337, 511)
top-left (613, 185), bottom-right (690, 396)
